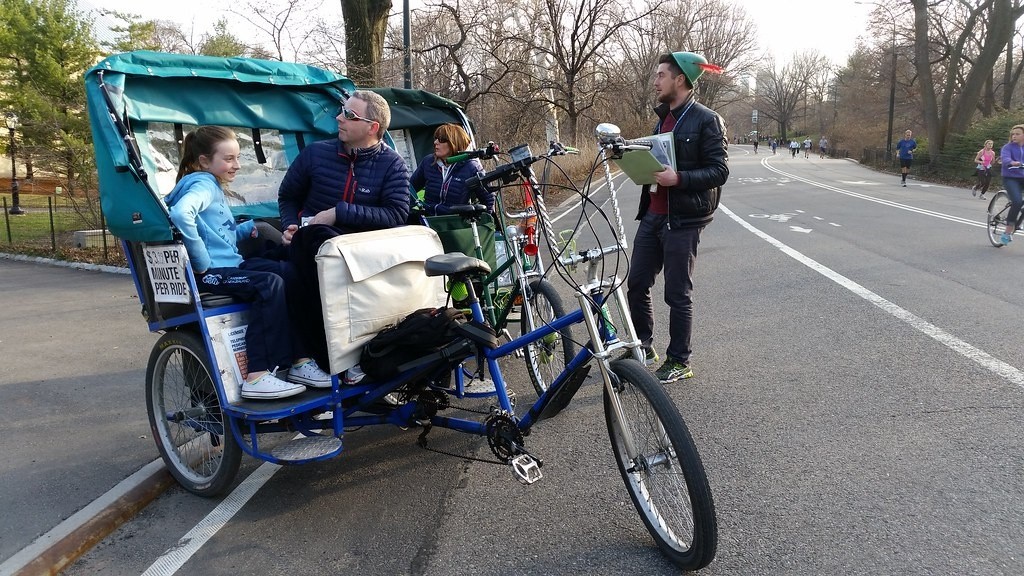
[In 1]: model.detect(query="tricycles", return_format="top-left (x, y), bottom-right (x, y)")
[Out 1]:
top-left (84, 51), bottom-right (717, 573)
top-left (350, 80), bottom-right (580, 401)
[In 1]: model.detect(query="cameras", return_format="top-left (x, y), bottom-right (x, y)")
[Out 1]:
top-left (301, 216), bottom-right (315, 227)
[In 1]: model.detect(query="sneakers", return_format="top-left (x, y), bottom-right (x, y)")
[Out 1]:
top-left (287, 359), bottom-right (341, 387)
top-left (241, 366), bottom-right (306, 399)
top-left (609, 338), bottom-right (659, 368)
top-left (902, 181), bottom-right (907, 187)
top-left (343, 365), bottom-right (367, 385)
top-left (655, 355), bottom-right (694, 383)
top-left (979, 194), bottom-right (986, 200)
top-left (1000, 232), bottom-right (1013, 246)
top-left (972, 186), bottom-right (977, 196)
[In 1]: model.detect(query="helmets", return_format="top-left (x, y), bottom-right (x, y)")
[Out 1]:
top-left (669, 47), bottom-right (725, 90)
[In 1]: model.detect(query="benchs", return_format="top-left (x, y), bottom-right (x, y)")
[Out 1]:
top-left (122, 200), bottom-right (282, 406)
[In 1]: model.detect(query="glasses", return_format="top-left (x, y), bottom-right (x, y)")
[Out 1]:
top-left (341, 106), bottom-right (374, 124)
top-left (434, 134), bottom-right (448, 144)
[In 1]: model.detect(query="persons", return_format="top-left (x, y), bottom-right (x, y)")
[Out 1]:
top-left (754, 140), bottom-right (759, 152)
top-left (787, 138), bottom-right (801, 158)
top-left (614, 52), bottom-right (730, 383)
top-left (411, 123), bottom-right (495, 215)
top-left (972, 140), bottom-right (995, 200)
top-left (768, 136), bottom-right (784, 155)
top-left (803, 136), bottom-right (812, 159)
top-left (1000, 125), bottom-right (1024, 244)
top-left (277, 90), bottom-right (410, 384)
top-left (163, 125), bottom-right (342, 400)
top-left (896, 129), bottom-right (917, 187)
top-left (819, 136), bottom-right (827, 159)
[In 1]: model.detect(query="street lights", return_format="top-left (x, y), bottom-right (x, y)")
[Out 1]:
top-left (855, 1), bottom-right (900, 163)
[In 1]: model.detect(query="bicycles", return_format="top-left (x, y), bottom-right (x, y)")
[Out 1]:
top-left (987, 163), bottom-right (1024, 249)
top-left (753, 144), bottom-right (758, 154)
